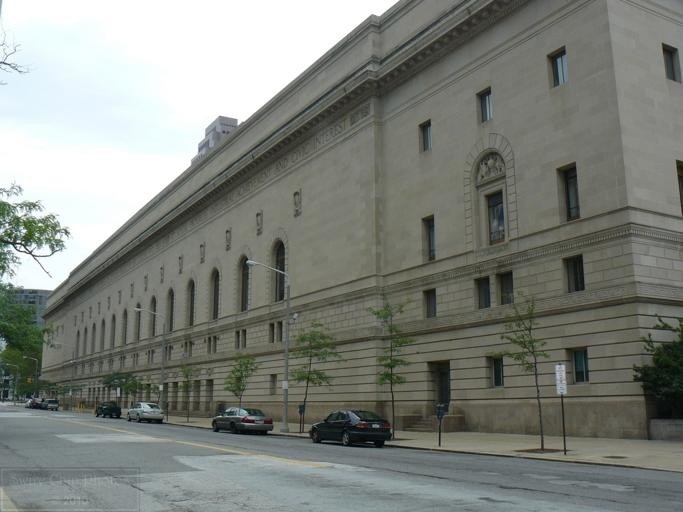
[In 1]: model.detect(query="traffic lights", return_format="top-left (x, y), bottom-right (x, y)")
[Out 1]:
top-left (26, 376), bottom-right (30, 384)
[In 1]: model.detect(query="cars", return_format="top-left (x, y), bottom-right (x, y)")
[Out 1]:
top-left (94, 401), bottom-right (122, 419)
top-left (24, 397), bottom-right (60, 412)
top-left (210, 406), bottom-right (275, 436)
top-left (306, 406), bottom-right (392, 449)
top-left (124, 401), bottom-right (164, 424)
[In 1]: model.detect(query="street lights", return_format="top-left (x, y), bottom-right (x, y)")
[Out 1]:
top-left (131, 305), bottom-right (166, 412)
top-left (54, 342), bottom-right (77, 413)
top-left (22, 355), bottom-right (38, 397)
top-left (243, 258), bottom-right (290, 434)
top-left (5, 362), bottom-right (19, 406)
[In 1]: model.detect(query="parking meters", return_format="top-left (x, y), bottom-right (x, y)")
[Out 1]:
top-left (434, 402), bottom-right (445, 448)
top-left (296, 403), bottom-right (306, 433)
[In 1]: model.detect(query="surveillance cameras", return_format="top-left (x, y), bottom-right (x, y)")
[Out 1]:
top-left (293, 313), bottom-right (298, 320)
